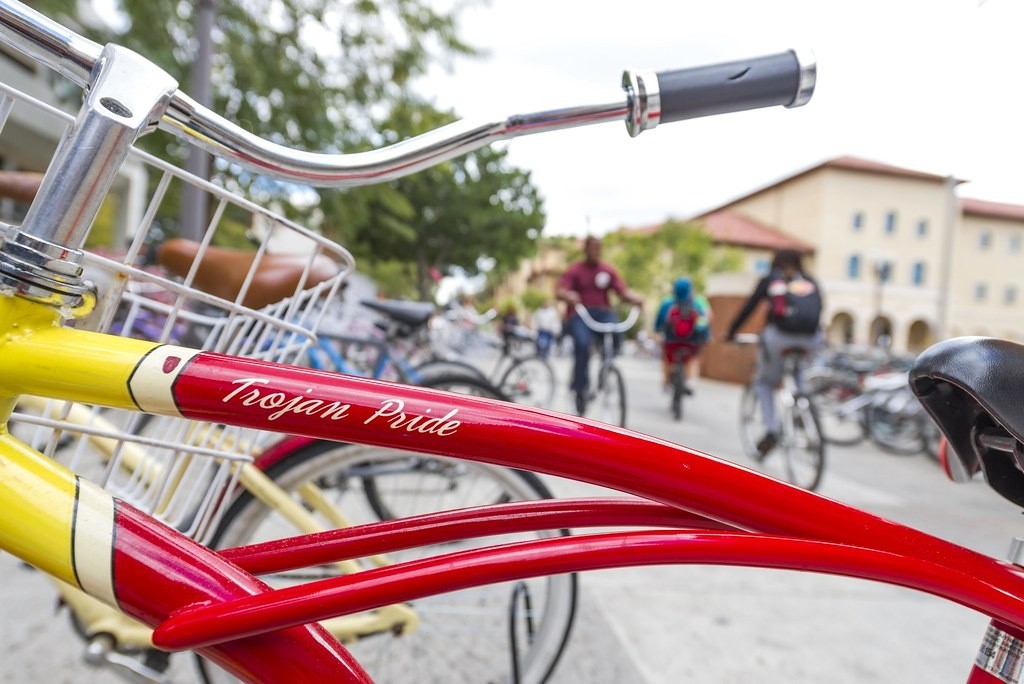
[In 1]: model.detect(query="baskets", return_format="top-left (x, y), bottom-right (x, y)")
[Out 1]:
top-left (0, 81), bottom-right (356, 545)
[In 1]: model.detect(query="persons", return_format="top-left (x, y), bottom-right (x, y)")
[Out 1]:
top-left (653, 276), bottom-right (716, 400)
top-left (554, 233), bottom-right (645, 416)
top-left (719, 247), bottom-right (826, 454)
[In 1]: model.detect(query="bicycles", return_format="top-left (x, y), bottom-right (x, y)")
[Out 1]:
top-left (718, 331), bottom-right (827, 492)
top-left (571, 297), bottom-right (643, 428)
top-left (0, 0), bottom-right (1024, 683)
top-left (656, 332), bottom-right (700, 419)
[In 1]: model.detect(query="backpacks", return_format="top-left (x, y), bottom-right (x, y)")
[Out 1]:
top-left (768, 267), bottom-right (821, 334)
top-left (669, 296), bottom-right (698, 339)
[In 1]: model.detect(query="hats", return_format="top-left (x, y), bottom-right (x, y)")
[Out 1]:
top-left (674, 279), bottom-right (691, 296)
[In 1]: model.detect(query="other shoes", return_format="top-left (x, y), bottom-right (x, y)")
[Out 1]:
top-left (756, 433), bottom-right (782, 452)
